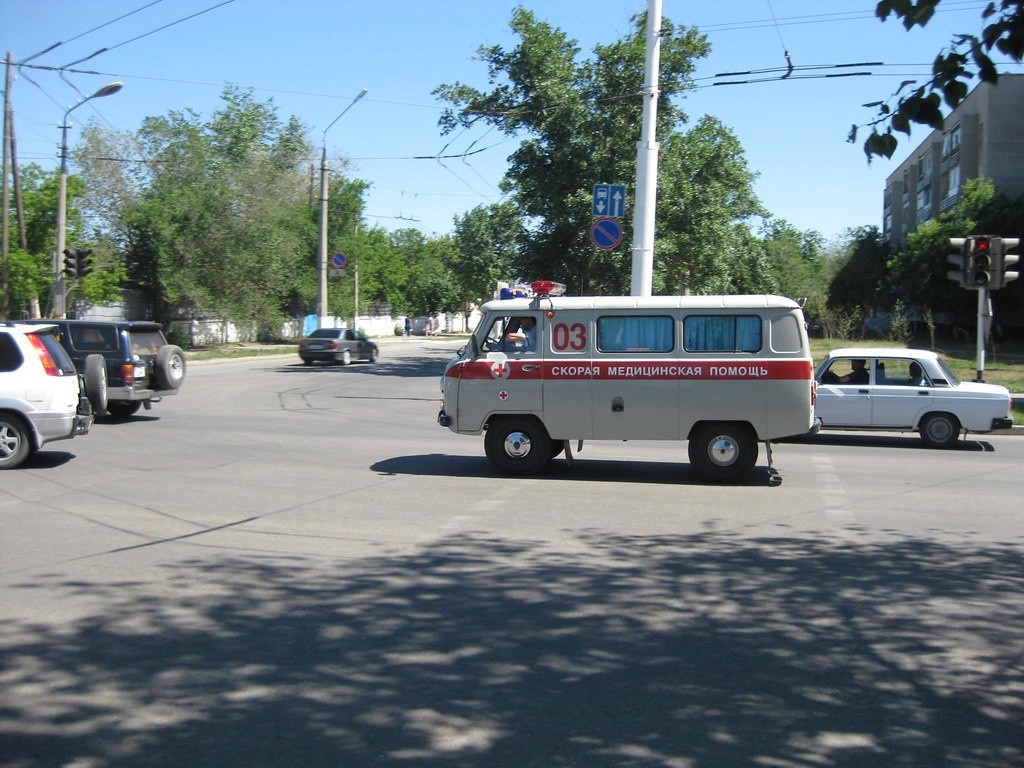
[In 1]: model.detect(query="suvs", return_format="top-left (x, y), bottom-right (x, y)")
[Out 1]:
top-left (0, 321), bottom-right (108, 470)
top-left (10, 319), bottom-right (187, 419)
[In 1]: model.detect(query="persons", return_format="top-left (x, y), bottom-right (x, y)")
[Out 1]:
top-left (405, 314), bottom-right (439, 337)
top-left (831, 360), bottom-right (869, 384)
top-left (906, 362), bottom-right (922, 386)
top-left (504, 318), bottom-right (536, 351)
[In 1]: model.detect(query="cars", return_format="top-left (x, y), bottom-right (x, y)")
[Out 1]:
top-left (297, 327), bottom-right (379, 365)
top-left (813, 347), bottom-right (1014, 448)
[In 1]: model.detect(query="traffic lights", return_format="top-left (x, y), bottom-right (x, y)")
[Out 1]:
top-left (999, 236), bottom-right (1021, 290)
top-left (946, 236), bottom-right (968, 288)
top-left (77, 246), bottom-right (94, 278)
top-left (966, 234), bottom-right (1003, 290)
top-left (62, 247), bottom-right (78, 277)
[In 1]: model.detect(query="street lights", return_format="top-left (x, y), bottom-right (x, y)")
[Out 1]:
top-left (47, 82), bottom-right (128, 315)
top-left (318, 88), bottom-right (371, 328)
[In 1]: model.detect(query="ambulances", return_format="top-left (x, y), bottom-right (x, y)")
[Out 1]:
top-left (436, 279), bottom-right (822, 483)
